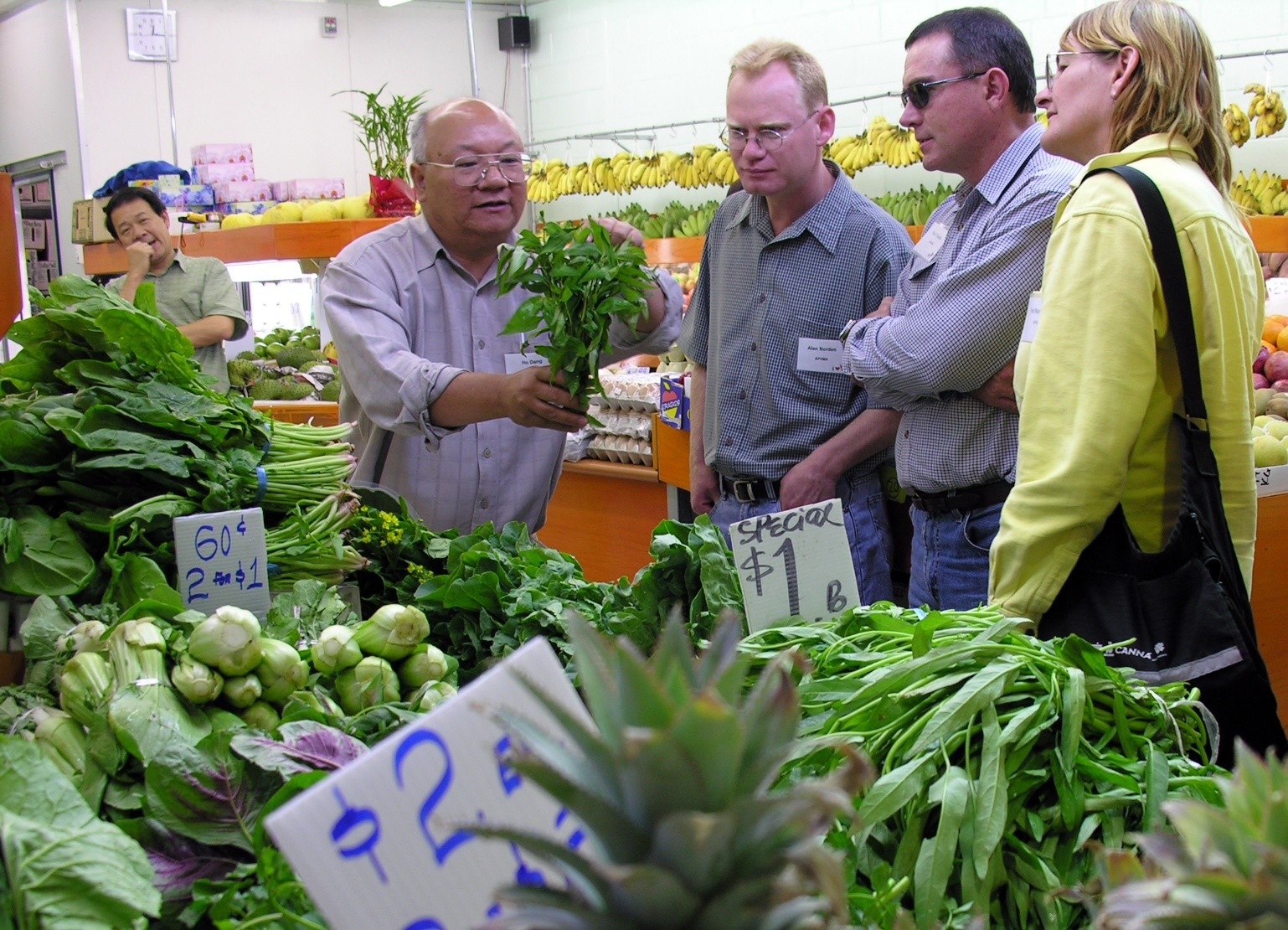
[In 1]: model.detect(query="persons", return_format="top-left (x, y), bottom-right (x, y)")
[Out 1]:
top-left (840, 6), bottom-right (1084, 611)
top-left (319, 98), bottom-right (685, 549)
top-left (674, 40), bottom-right (915, 606)
top-left (988, 0), bottom-right (1266, 638)
top-left (104, 186), bottom-right (248, 398)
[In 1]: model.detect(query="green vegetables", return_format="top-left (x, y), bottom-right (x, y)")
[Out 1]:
top-left (495, 215), bottom-right (663, 430)
top-left (0, 490), bottom-right (1231, 930)
top-left (0, 274), bottom-right (356, 513)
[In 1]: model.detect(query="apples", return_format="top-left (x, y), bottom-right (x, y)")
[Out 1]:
top-left (671, 262), bottom-right (700, 314)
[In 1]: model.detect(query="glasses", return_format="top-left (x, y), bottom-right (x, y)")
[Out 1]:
top-left (419, 152), bottom-right (535, 186)
top-left (1044, 49), bottom-right (1139, 90)
top-left (719, 109), bottom-right (820, 151)
top-left (901, 69), bottom-right (1012, 109)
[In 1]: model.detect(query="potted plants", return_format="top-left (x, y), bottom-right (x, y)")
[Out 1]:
top-left (331, 82), bottom-right (433, 219)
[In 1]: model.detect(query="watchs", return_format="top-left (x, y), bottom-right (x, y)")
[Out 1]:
top-left (838, 319), bottom-right (861, 351)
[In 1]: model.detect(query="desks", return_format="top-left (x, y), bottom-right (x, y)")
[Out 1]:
top-left (546, 459), bottom-right (681, 584)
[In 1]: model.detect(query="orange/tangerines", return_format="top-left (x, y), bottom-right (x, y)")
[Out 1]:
top-left (1260, 314), bottom-right (1288, 353)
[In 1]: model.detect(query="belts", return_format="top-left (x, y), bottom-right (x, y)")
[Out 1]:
top-left (908, 486), bottom-right (1013, 516)
top-left (716, 468), bottom-right (787, 503)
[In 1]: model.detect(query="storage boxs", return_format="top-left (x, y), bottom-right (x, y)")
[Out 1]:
top-left (649, 411), bottom-right (693, 495)
top-left (656, 374), bottom-right (693, 429)
top-left (19, 137), bottom-right (347, 317)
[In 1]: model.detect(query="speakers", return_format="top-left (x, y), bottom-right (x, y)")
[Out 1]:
top-left (498, 16), bottom-right (530, 49)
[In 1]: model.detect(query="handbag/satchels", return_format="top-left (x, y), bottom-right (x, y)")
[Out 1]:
top-left (1038, 412), bottom-right (1287, 777)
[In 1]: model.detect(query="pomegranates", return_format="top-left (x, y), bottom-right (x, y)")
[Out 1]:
top-left (1252, 345), bottom-right (1288, 393)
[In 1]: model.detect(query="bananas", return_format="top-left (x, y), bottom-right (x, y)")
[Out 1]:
top-left (1228, 166), bottom-right (1288, 217)
top-left (823, 111), bottom-right (1050, 226)
top-left (523, 144), bottom-right (741, 238)
top-left (1219, 83), bottom-right (1287, 146)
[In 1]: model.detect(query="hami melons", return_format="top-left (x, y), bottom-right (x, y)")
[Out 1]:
top-left (219, 190), bottom-right (372, 231)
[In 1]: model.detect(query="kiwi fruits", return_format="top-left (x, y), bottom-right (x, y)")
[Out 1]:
top-left (1253, 388), bottom-right (1288, 420)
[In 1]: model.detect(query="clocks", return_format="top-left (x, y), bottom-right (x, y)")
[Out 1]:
top-left (125, 7), bottom-right (178, 63)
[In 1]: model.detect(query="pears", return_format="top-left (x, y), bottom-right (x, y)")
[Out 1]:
top-left (1251, 415), bottom-right (1288, 468)
